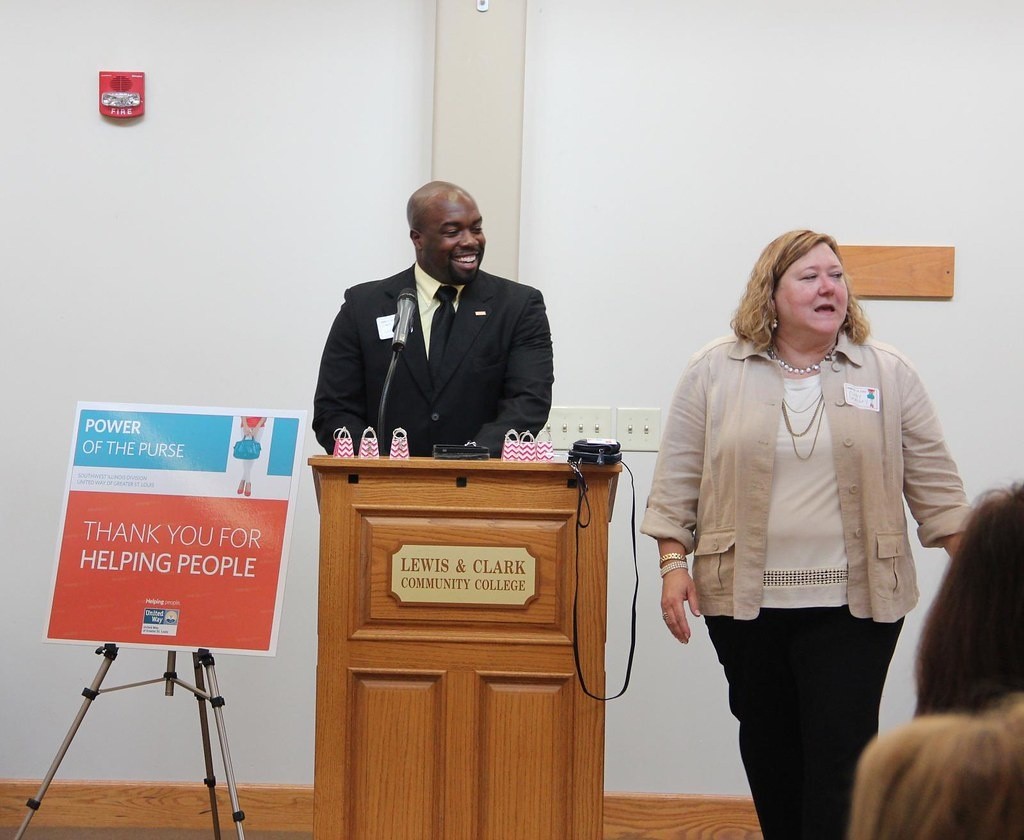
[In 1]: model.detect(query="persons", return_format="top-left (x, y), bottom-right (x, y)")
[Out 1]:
top-left (236, 416), bottom-right (267, 497)
top-left (639, 230), bottom-right (973, 840)
top-left (849, 484), bottom-right (1023, 840)
top-left (312, 180), bottom-right (554, 460)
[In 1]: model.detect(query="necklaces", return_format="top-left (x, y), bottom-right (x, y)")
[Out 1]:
top-left (781, 390), bottom-right (825, 461)
top-left (767, 343), bottom-right (835, 373)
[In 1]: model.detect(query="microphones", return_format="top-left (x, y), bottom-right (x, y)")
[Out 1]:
top-left (392, 288), bottom-right (418, 351)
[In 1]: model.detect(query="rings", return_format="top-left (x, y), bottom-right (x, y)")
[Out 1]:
top-left (663, 613), bottom-right (669, 621)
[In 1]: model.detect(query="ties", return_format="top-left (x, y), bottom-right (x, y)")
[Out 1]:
top-left (428, 285), bottom-right (458, 389)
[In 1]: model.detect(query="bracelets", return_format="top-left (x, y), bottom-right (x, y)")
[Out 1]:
top-left (659, 552), bottom-right (689, 578)
